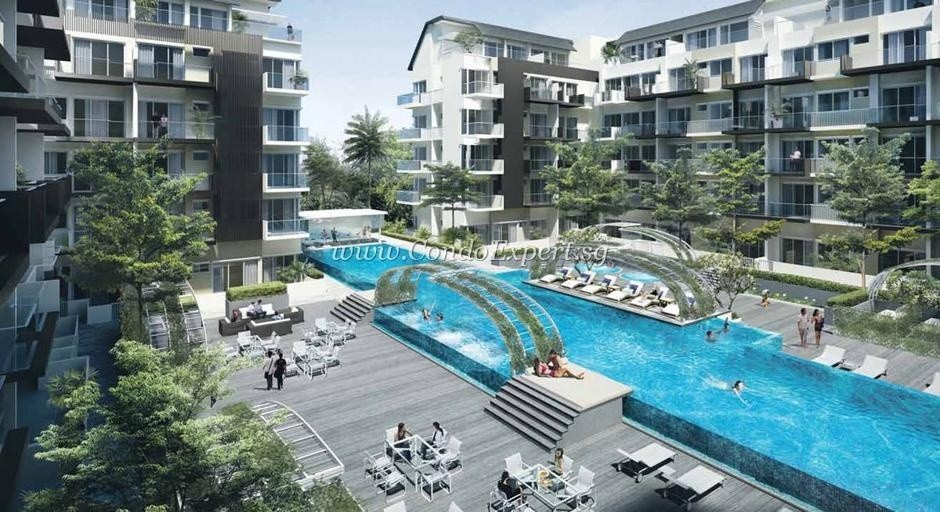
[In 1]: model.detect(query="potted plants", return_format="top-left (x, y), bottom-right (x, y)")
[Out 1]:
top-left (682, 58), bottom-right (697, 89)
top-left (602, 40), bottom-right (625, 65)
top-left (767, 100), bottom-right (793, 129)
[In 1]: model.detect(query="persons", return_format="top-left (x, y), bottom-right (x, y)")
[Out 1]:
top-left (697, 371), bottom-right (767, 408)
top-left (721, 321), bottom-right (730, 334)
top-left (360, 226), bottom-right (372, 239)
top-left (230, 299), bottom-right (282, 324)
top-left (704, 330), bottom-right (719, 341)
top-left (534, 350), bottom-right (585, 380)
top-left (543, 447), bottom-right (565, 493)
top-left (262, 349), bottom-right (287, 391)
top-left (759, 288), bottom-right (771, 309)
top-left (394, 423), bottom-right (413, 464)
top-left (812, 309), bottom-right (824, 347)
top-left (421, 422), bottom-right (444, 458)
top-left (421, 309), bottom-right (444, 322)
top-left (796, 308), bottom-right (810, 349)
top-left (321, 226), bottom-right (338, 244)
top-left (498, 471), bottom-right (527, 504)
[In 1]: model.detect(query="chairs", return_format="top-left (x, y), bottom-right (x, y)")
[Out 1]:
top-left (851, 353), bottom-right (888, 381)
top-left (922, 371), bottom-right (940, 398)
top-left (217, 304), bottom-right (358, 382)
top-left (614, 440), bottom-right (677, 482)
top-left (810, 345), bottom-right (845, 369)
top-left (538, 266), bottom-right (696, 317)
top-left (360, 426), bottom-right (598, 511)
top-left (661, 461), bottom-right (726, 507)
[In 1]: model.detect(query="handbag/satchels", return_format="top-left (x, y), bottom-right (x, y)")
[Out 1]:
top-left (264, 372), bottom-right (268, 378)
top-left (274, 370), bottom-right (278, 377)
